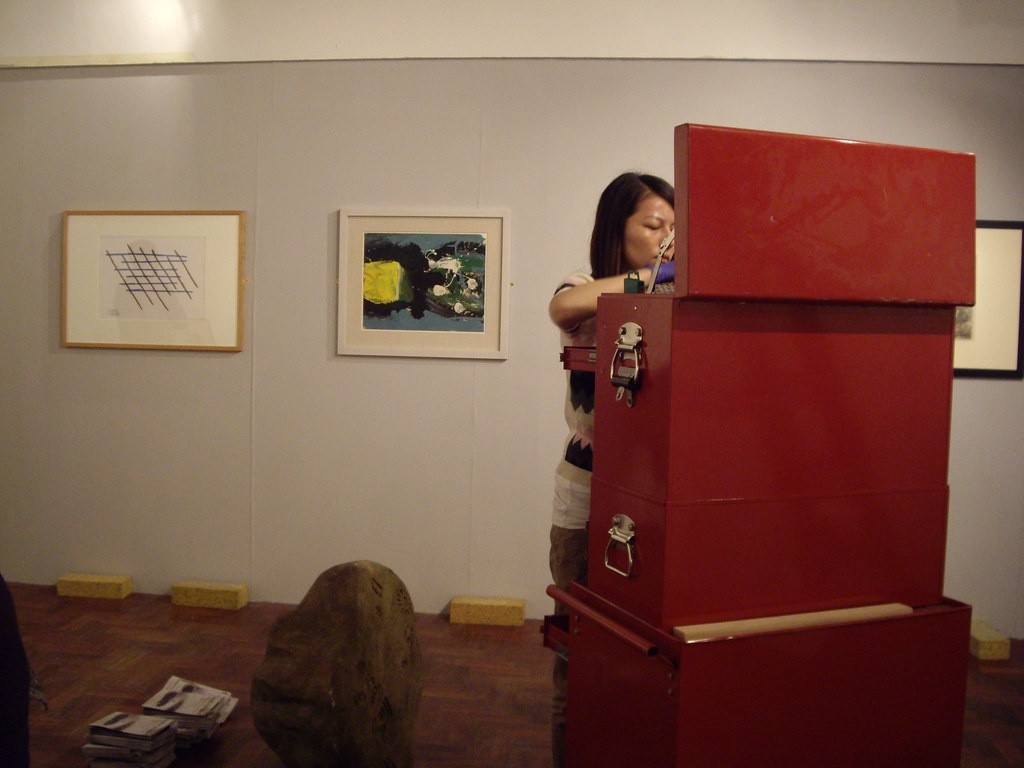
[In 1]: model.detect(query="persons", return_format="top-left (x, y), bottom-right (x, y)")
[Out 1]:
top-left (549, 172), bottom-right (674, 768)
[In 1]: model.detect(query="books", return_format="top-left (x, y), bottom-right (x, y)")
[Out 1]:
top-left (81, 673), bottom-right (241, 768)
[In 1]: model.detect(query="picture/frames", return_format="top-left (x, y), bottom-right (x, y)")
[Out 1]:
top-left (61, 211), bottom-right (245, 351)
top-left (953, 220), bottom-right (1024, 379)
top-left (337, 209), bottom-right (511, 360)
